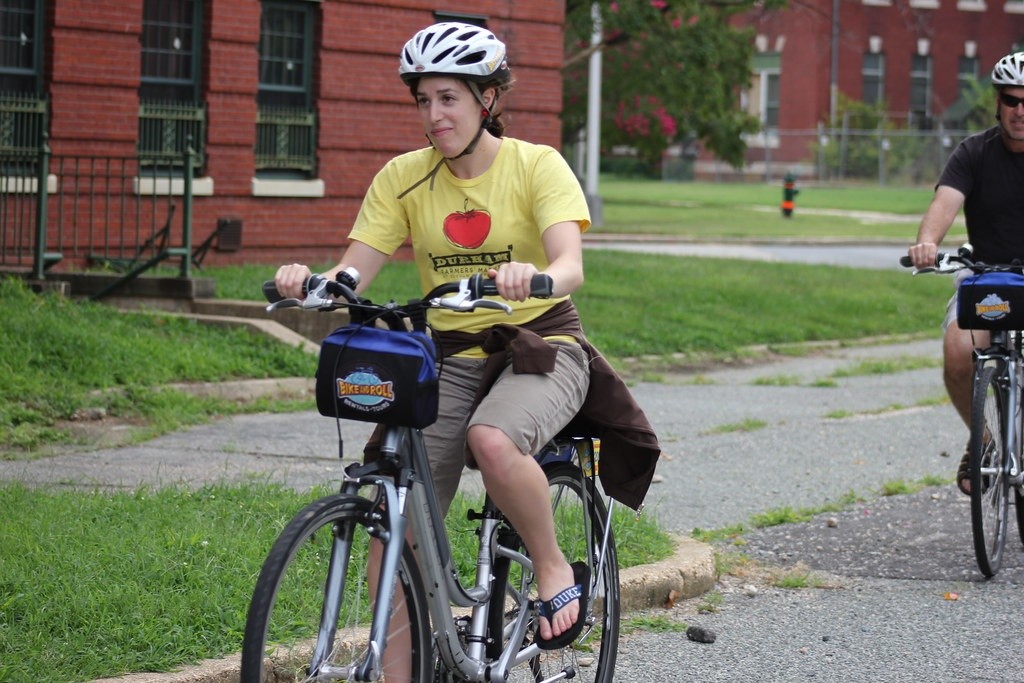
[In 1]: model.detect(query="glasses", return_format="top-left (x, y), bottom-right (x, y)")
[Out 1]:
top-left (999, 91), bottom-right (1024, 107)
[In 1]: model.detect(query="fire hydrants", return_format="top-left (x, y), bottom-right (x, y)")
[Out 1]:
top-left (779, 173), bottom-right (799, 219)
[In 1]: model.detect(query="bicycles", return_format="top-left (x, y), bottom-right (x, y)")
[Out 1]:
top-left (899, 242), bottom-right (1024, 578)
top-left (240, 267), bottom-right (621, 683)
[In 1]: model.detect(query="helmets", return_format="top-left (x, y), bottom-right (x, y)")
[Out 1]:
top-left (397, 22), bottom-right (509, 90)
top-left (990, 52), bottom-right (1024, 87)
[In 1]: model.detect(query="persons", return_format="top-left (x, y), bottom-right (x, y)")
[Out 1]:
top-left (908, 49), bottom-right (1023, 495)
top-left (275, 23), bottom-right (596, 683)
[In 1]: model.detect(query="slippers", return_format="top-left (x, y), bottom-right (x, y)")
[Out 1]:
top-left (956, 434), bottom-right (996, 498)
top-left (536, 561), bottom-right (593, 649)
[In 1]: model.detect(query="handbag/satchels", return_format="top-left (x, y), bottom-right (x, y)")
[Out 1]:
top-left (958, 272), bottom-right (1023, 331)
top-left (313, 323), bottom-right (440, 429)
